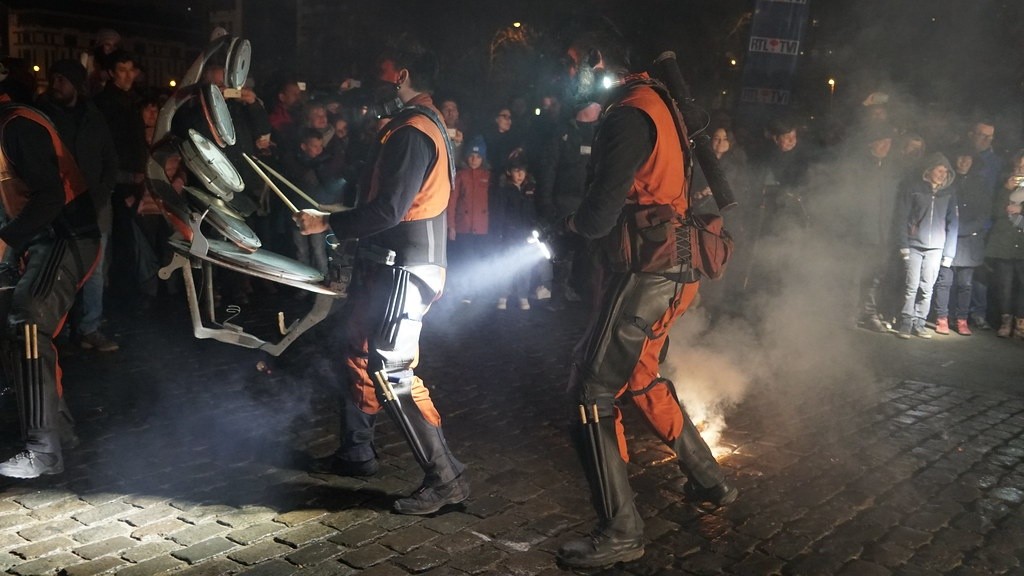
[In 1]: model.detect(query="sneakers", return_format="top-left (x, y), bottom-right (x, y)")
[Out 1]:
top-left (59, 434), bottom-right (80, 449)
top-left (0, 448), bottom-right (64, 478)
top-left (557, 529), bottom-right (645, 567)
top-left (682, 475), bottom-right (738, 505)
top-left (393, 475), bottom-right (470, 515)
top-left (308, 452), bottom-right (379, 476)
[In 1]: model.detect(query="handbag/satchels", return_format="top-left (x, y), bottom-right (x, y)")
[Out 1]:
top-left (687, 211), bottom-right (734, 281)
top-left (611, 204), bottom-right (679, 275)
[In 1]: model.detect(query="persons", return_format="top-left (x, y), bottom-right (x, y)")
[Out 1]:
top-left (0, 101), bottom-right (101, 477)
top-left (427, 69), bottom-right (607, 305)
top-left (666, 50), bottom-right (1024, 339)
top-left (291, 31), bottom-right (471, 514)
top-left (0, 27), bottom-right (375, 355)
top-left (528, 10), bottom-right (736, 576)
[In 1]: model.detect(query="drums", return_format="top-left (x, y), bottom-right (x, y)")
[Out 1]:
top-left (168, 232), bottom-right (325, 284)
top-left (200, 82), bottom-right (237, 149)
top-left (180, 192), bottom-right (262, 253)
top-left (222, 36), bottom-right (252, 91)
top-left (178, 128), bottom-right (246, 202)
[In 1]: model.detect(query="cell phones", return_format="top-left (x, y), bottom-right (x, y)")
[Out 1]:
top-left (298, 82), bottom-right (306, 91)
top-left (350, 80), bottom-right (360, 87)
top-left (223, 89), bottom-right (241, 98)
top-left (1013, 176), bottom-right (1024, 186)
top-left (447, 129), bottom-right (456, 137)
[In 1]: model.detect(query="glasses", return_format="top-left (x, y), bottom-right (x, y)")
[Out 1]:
top-left (496, 113), bottom-right (512, 120)
top-left (970, 131), bottom-right (993, 143)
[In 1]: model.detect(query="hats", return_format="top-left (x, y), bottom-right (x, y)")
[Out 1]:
top-left (465, 134), bottom-right (488, 163)
top-left (93, 27), bottom-right (123, 48)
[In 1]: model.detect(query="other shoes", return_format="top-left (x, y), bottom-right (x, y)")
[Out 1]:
top-left (954, 318), bottom-right (971, 334)
top-left (936, 316), bottom-right (950, 335)
top-left (82, 330), bottom-right (118, 352)
top-left (496, 295), bottom-right (506, 309)
top-left (461, 292), bottom-right (472, 303)
top-left (534, 284), bottom-right (551, 300)
top-left (911, 322), bottom-right (932, 339)
top-left (865, 314), bottom-right (885, 333)
top-left (1015, 319), bottom-right (1024, 337)
top-left (997, 316), bottom-right (1013, 337)
top-left (973, 317), bottom-right (987, 330)
top-left (898, 322), bottom-right (912, 338)
top-left (519, 296), bottom-right (530, 310)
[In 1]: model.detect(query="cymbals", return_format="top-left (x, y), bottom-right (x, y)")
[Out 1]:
top-left (183, 186), bottom-right (245, 221)
